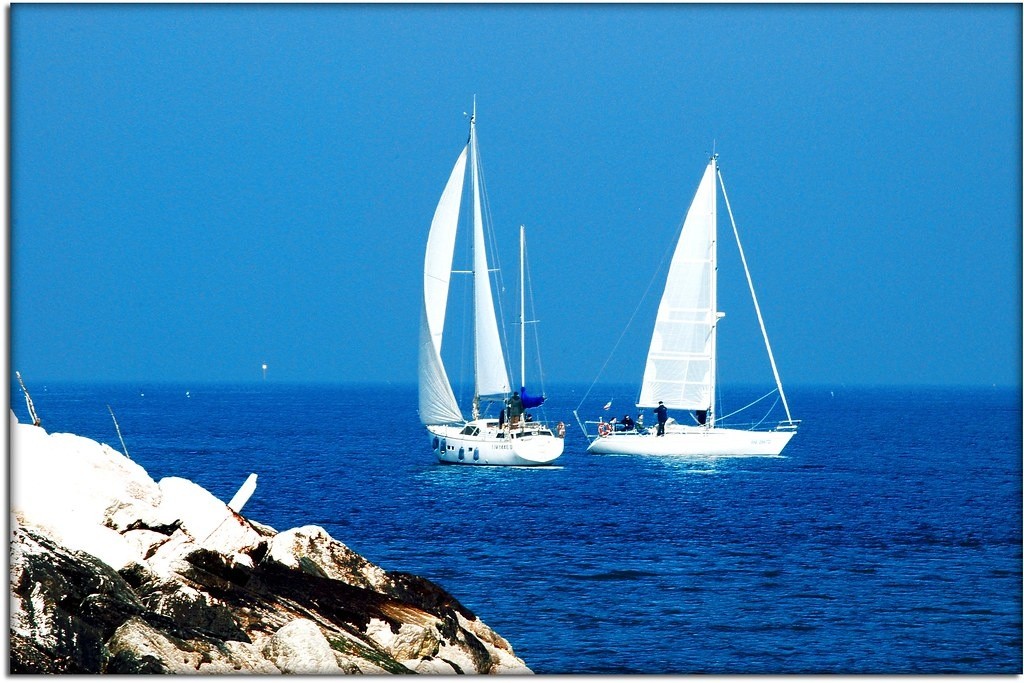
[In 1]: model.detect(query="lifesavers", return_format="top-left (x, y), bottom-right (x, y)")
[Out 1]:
top-left (557, 422), bottom-right (566, 438)
top-left (597, 422), bottom-right (611, 438)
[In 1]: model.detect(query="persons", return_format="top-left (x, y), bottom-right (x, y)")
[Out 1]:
top-left (654, 401), bottom-right (667, 437)
top-left (507, 392), bottom-right (533, 428)
top-left (610, 414), bottom-right (653, 435)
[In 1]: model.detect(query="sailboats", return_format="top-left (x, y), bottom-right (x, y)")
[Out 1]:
top-left (573, 137), bottom-right (802, 457)
top-left (417, 93), bottom-right (565, 465)
